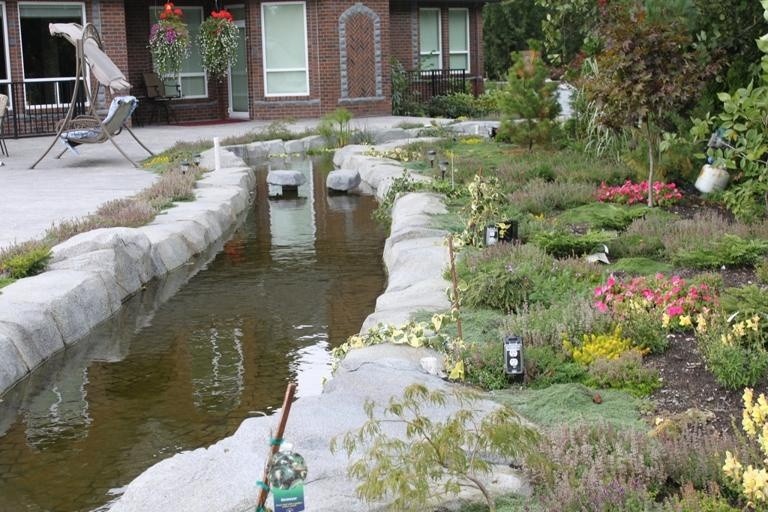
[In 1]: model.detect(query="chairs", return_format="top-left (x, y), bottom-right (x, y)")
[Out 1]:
top-left (1, 93), bottom-right (11, 157)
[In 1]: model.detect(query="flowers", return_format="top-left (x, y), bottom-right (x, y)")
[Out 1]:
top-left (196, 9), bottom-right (241, 84)
top-left (147, 0), bottom-right (193, 83)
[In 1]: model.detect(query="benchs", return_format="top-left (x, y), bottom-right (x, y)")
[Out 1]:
top-left (26, 20), bottom-right (157, 170)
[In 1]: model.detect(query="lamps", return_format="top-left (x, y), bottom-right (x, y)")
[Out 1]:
top-left (502, 335), bottom-right (524, 376)
top-left (439, 161), bottom-right (450, 181)
top-left (426, 150), bottom-right (437, 169)
top-left (483, 225), bottom-right (498, 247)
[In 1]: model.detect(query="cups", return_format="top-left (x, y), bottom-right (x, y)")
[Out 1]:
top-left (419, 356), bottom-right (438, 375)
